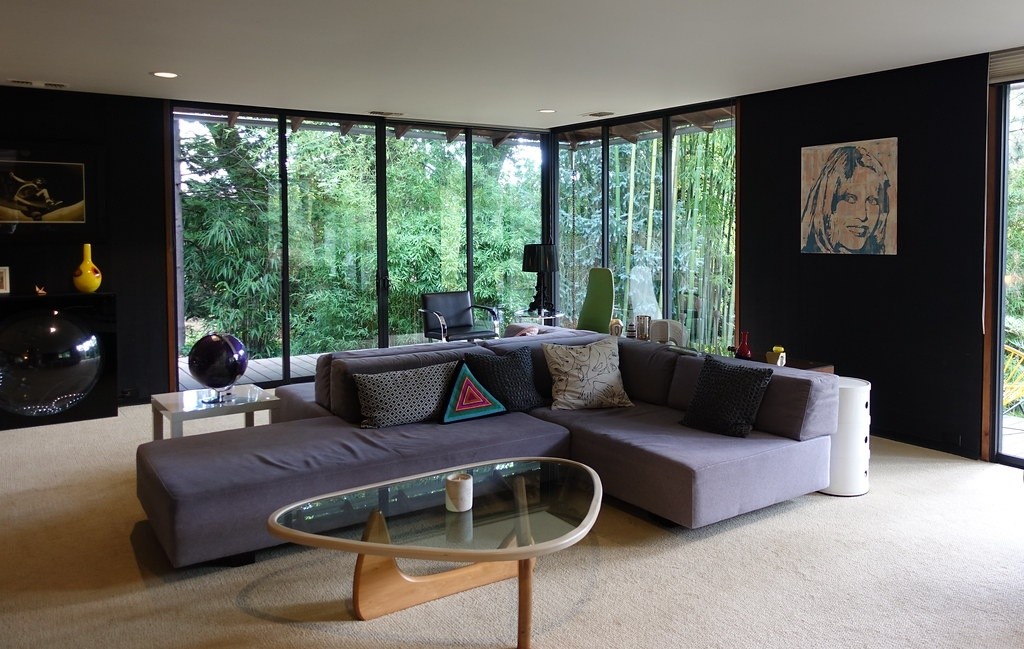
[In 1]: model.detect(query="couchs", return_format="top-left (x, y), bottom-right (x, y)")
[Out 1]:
top-left (136, 326), bottom-right (841, 570)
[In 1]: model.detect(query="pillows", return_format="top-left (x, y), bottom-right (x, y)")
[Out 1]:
top-left (464, 348), bottom-right (539, 413)
top-left (352, 358), bottom-right (462, 428)
top-left (437, 358), bottom-right (504, 425)
top-left (679, 354), bottom-right (775, 435)
top-left (542, 337), bottom-right (633, 410)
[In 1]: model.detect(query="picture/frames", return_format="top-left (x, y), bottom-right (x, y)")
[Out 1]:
top-left (0, 143), bottom-right (109, 245)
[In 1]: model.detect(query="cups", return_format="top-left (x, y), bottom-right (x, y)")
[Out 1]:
top-left (446, 474), bottom-right (473, 512)
top-left (636, 316), bottom-right (651, 340)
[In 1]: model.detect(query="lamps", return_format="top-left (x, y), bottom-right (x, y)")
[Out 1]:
top-left (522, 244), bottom-right (559, 325)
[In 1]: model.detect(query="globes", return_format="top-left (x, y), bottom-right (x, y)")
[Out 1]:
top-left (189, 333), bottom-right (249, 404)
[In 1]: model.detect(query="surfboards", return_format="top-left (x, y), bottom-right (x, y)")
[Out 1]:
top-left (575, 268), bottom-right (615, 334)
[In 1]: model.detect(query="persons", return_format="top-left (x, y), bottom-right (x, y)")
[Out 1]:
top-left (9, 170), bottom-right (64, 223)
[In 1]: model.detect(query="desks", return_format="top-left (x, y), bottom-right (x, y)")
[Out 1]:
top-left (151, 383), bottom-right (279, 441)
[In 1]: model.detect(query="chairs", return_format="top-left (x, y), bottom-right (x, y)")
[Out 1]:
top-left (419, 291), bottom-right (501, 342)
top-left (576, 267), bottom-right (615, 334)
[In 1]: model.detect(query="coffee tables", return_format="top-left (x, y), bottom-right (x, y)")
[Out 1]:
top-left (269, 452), bottom-right (603, 649)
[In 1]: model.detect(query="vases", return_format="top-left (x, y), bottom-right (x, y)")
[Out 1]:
top-left (73, 243), bottom-right (101, 293)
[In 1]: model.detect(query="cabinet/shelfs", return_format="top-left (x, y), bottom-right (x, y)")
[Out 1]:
top-left (0, 292), bottom-right (117, 430)
